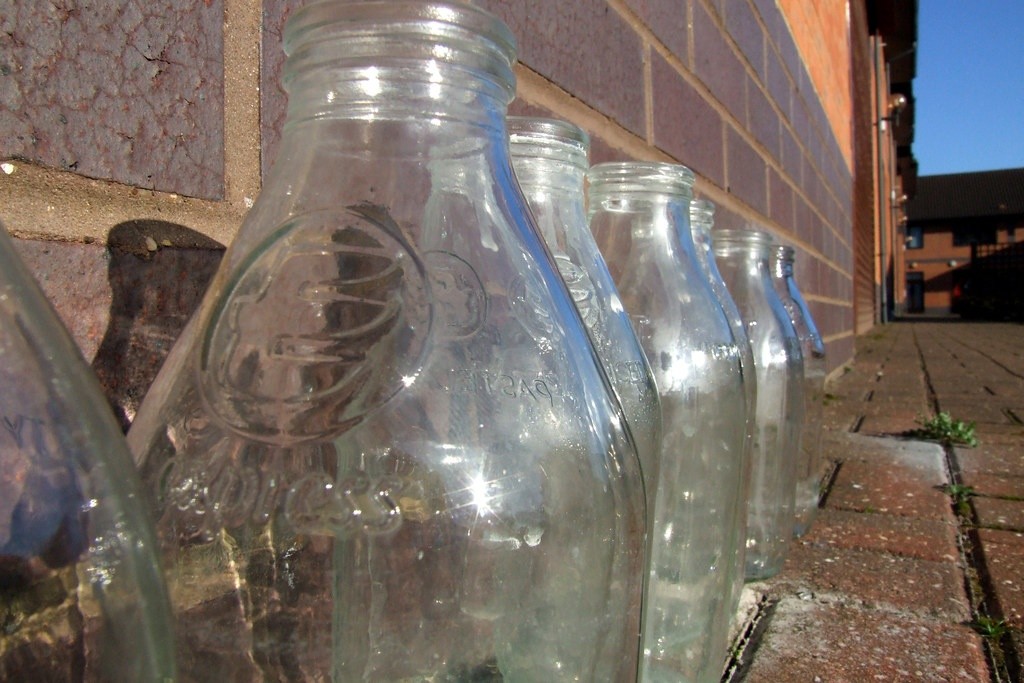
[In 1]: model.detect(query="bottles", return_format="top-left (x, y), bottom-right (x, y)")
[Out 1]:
top-left (0, 0), bottom-right (826, 683)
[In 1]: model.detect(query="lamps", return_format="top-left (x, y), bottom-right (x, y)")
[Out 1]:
top-left (881, 94), bottom-right (907, 127)
